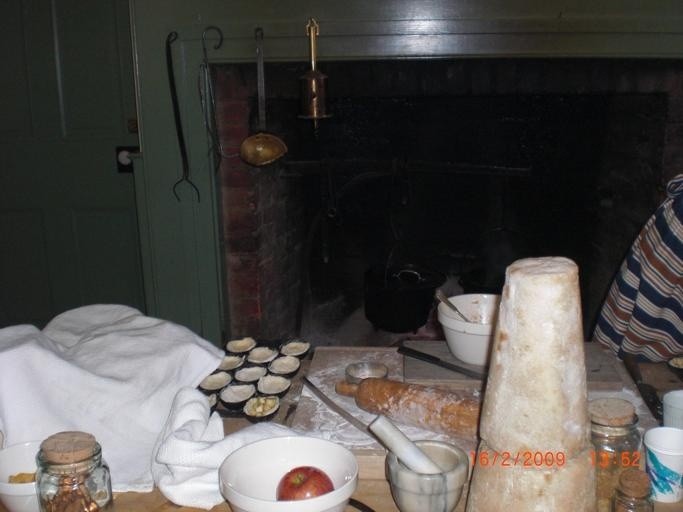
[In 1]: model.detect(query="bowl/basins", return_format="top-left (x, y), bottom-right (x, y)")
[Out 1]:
top-left (0, 442), bottom-right (39, 511)
top-left (438, 294), bottom-right (500, 366)
top-left (199, 336), bottom-right (361, 511)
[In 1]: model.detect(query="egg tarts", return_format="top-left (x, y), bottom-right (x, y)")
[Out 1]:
top-left (200, 336), bottom-right (310, 417)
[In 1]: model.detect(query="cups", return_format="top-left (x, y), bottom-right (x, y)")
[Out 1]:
top-left (643, 390), bottom-right (683, 506)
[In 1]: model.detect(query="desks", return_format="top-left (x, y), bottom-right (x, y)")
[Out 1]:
top-left (110, 334), bottom-right (683, 509)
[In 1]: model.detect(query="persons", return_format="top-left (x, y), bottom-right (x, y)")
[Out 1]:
top-left (593, 172), bottom-right (683, 360)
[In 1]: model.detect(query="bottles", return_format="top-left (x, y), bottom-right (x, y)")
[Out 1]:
top-left (35, 430), bottom-right (113, 512)
top-left (589, 398), bottom-right (653, 512)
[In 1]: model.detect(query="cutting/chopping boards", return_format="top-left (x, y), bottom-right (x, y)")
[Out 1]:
top-left (288, 339), bottom-right (660, 480)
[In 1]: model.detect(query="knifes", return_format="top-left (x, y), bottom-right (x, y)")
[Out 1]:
top-left (617, 347), bottom-right (663, 427)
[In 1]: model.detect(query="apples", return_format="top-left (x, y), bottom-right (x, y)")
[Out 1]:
top-left (276, 465), bottom-right (334, 499)
